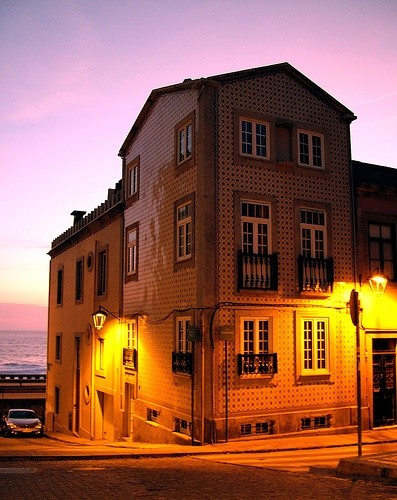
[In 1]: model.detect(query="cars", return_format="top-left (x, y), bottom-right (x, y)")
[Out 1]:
top-left (1, 409), bottom-right (43, 437)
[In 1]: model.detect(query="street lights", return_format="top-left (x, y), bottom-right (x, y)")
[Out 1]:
top-left (350, 267), bottom-right (388, 456)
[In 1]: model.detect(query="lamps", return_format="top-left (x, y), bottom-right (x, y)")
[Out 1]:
top-left (90, 304), bottom-right (118, 330)
top-left (359, 266), bottom-right (390, 296)
